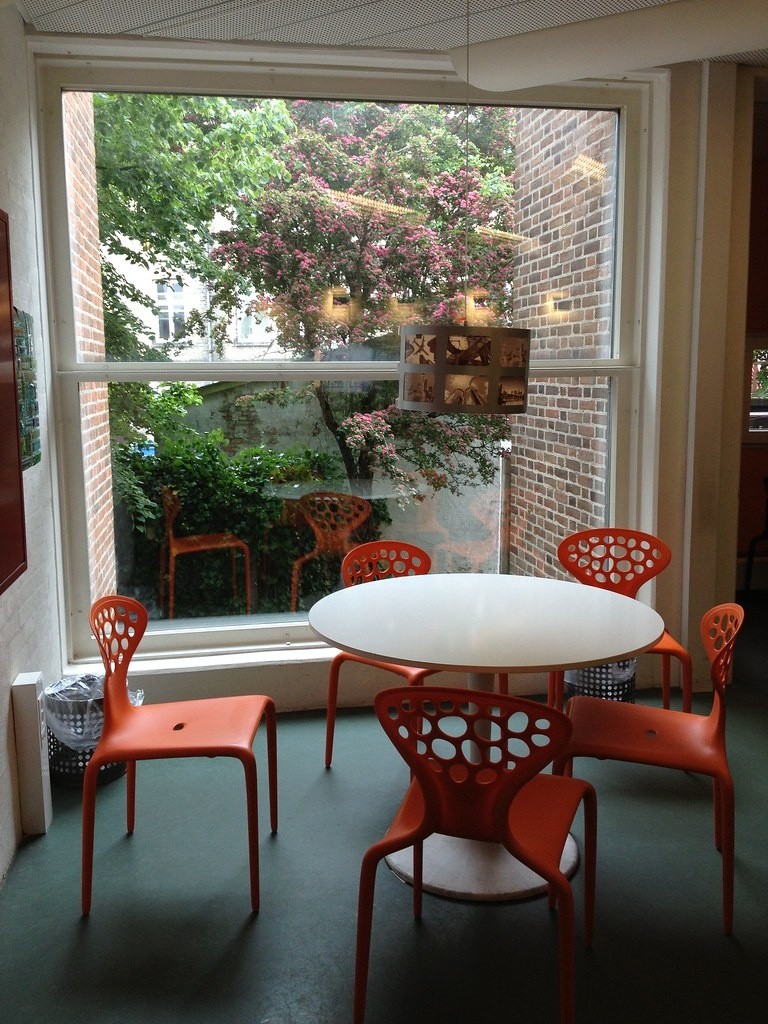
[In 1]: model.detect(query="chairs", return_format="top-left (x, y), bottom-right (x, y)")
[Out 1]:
top-left (552, 527), bottom-right (693, 717)
top-left (351, 686), bottom-right (600, 1024)
top-left (155, 485), bottom-right (252, 623)
top-left (325, 540), bottom-right (509, 784)
top-left (81, 594), bottom-right (279, 917)
top-left (290, 491), bottom-right (379, 613)
top-left (555, 601), bottom-right (746, 936)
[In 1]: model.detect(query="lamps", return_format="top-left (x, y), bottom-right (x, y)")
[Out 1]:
top-left (396, 0), bottom-right (532, 415)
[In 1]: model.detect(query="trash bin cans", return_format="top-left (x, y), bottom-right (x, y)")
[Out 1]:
top-left (565, 657), bottom-right (636, 705)
top-left (43, 673), bottom-right (127, 786)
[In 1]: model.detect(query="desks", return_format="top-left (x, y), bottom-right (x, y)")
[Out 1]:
top-left (260, 478), bottom-right (417, 502)
top-left (306, 573), bottom-right (665, 903)
